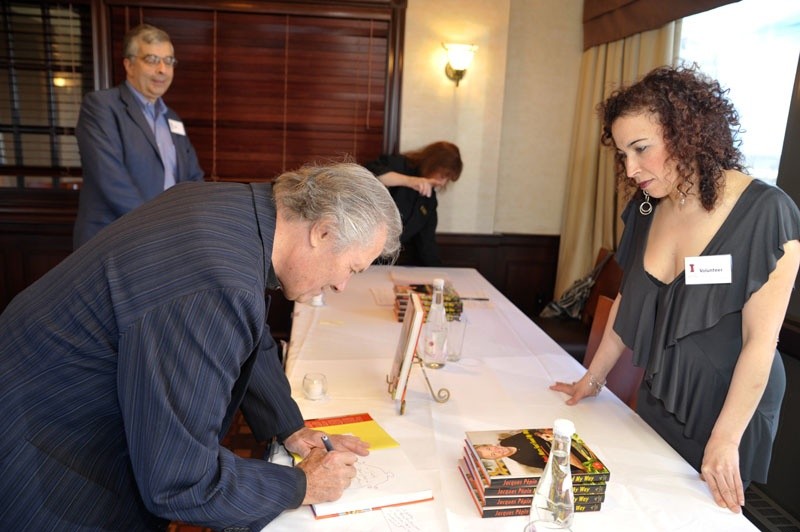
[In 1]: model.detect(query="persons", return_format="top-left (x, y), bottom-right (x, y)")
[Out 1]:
top-left (548, 59), bottom-right (800, 515)
top-left (71, 27), bottom-right (207, 252)
top-left (0, 161), bottom-right (404, 532)
top-left (368, 143), bottom-right (463, 269)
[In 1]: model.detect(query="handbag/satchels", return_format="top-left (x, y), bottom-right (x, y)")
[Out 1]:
top-left (559, 250), bottom-right (615, 319)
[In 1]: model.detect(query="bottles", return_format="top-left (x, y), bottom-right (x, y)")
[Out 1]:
top-left (423, 277), bottom-right (447, 369)
top-left (528, 418), bottom-right (575, 531)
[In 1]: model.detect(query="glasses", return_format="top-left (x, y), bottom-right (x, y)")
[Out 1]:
top-left (129, 54), bottom-right (177, 65)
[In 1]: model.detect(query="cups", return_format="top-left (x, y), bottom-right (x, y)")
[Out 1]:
top-left (303, 373), bottom-right (326, 399)
top-left (524, 519), bottom-right (571, 531)
top-left (444, 315), bottom-right (468, 363)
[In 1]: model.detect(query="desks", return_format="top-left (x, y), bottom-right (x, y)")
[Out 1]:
top-left (256, 266), bottom-right (760, 532)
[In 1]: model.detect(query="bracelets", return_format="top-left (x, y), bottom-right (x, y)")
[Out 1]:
top-left (581, 374), bottom-right (598, 390)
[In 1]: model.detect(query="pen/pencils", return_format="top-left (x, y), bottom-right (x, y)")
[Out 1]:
top-left (321, 435), bottom-right (333, 451)
top-left (460, 297), bottom-right (489, 301)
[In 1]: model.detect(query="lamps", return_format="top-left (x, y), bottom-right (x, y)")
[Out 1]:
top-left (440, 41), bottom-right (477, 87)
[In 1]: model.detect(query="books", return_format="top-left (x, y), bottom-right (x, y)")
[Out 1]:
top-left (393, 282), bottom-right (464, 324)
top-left (290, 412), bottom-right (434, 518)
top-left (458, 427), bottom-right (611, 518)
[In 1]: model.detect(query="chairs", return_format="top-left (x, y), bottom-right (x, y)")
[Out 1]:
top-left (542, 248), bottom-right (643, 411)
